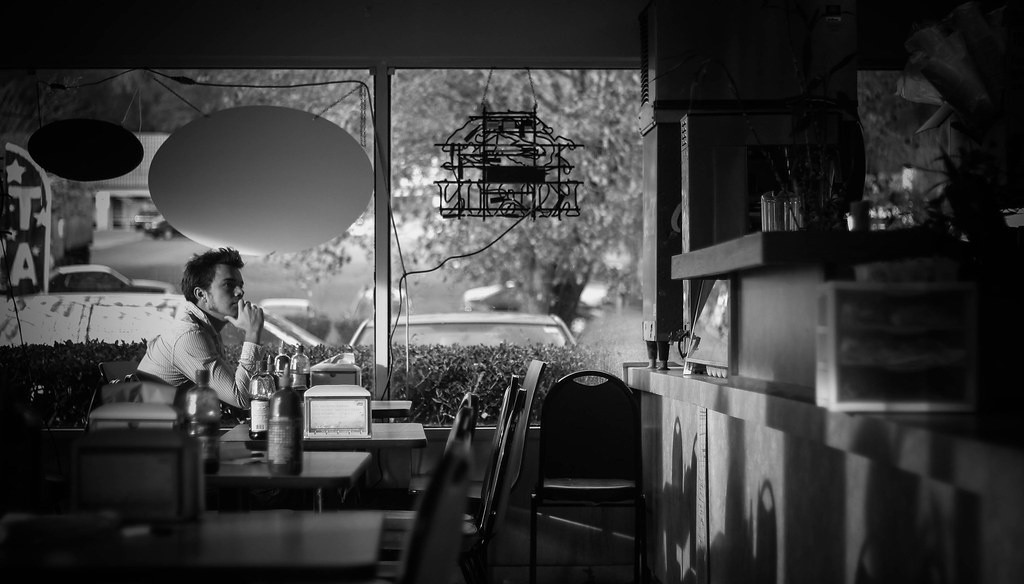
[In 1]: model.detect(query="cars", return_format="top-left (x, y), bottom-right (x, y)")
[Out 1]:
top-left (349, 312), bottom-right (577, 352)
top-left (45, 265), bottom-right (174, 292)
top-left (257, 296), bottom-right (316, 335)
top-left (132, 210), bottom-right (185, 242)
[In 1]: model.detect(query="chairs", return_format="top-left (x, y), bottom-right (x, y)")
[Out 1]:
top-left (530, 370), bottom-right (644, 584)
top-left (375, 373), bottom-right (527, 584)
top-left (98, 357), bottom-right (135, 385)
top-left (70, 430), bottom-right (202, 515)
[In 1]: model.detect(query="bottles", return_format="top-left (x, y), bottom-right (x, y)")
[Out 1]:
top-left (267, 363), bottom-right (303, 476)
top-left (184, 370), bottom-right (220, 475)
top-left (273, 340), bottom-right (309, 390)
top-left (249, 355), bottom-right (279, 439)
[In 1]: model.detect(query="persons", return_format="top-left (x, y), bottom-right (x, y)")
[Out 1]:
top-left (689, 280), bottom-right (730, 361)
top-left (136, 246), bottom-right (264, 409)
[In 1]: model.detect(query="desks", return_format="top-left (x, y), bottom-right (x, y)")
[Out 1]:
top-left (220, 417), bottom-right (430, 450)
top-left (302, 401), bottom-right (412, 417)
top-left (204, 449), bottom-right (371, 514)
top-left (0, 508), bottom-right (385, 584)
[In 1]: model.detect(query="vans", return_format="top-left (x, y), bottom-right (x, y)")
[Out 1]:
top-left (0, 289), bottom-right (304, 363)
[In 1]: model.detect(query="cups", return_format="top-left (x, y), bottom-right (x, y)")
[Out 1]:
top-left (761, 191), bottom-right (801, 232)
